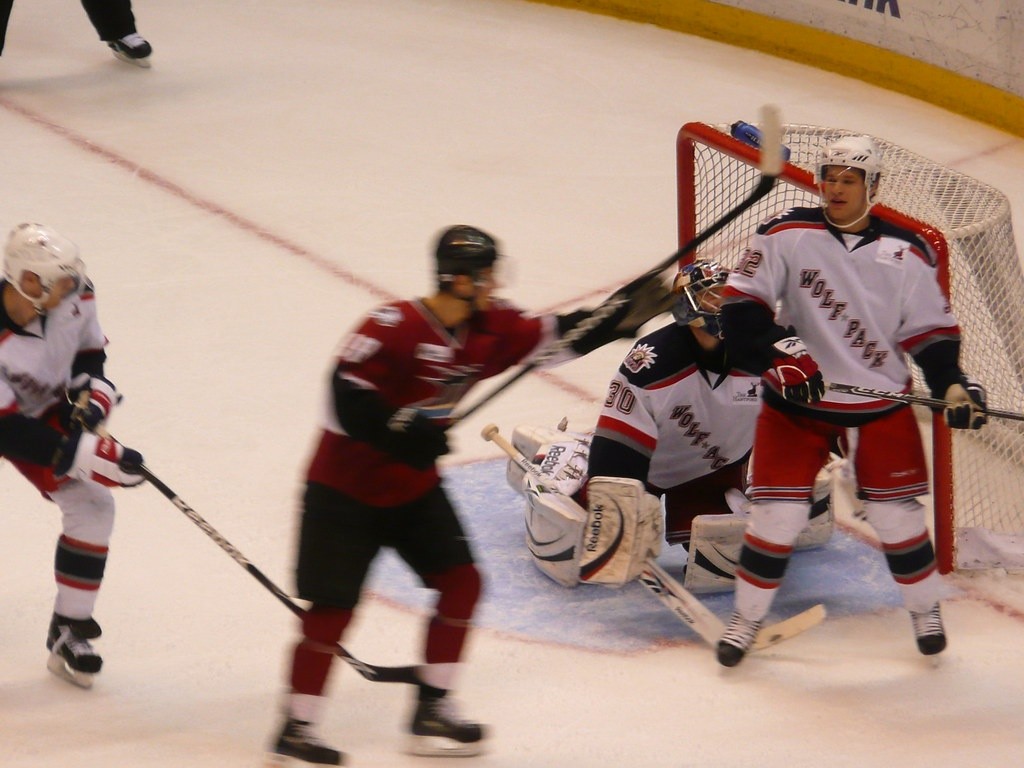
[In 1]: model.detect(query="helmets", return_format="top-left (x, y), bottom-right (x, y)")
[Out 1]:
top-left (435, 224), bottom-right (497, 281)
top-left (0, 222), bottom-right (81, 306)
top-left (813, 136), bottom-right (885, 189)
top-left (673, 258), bottom-right (732, 339)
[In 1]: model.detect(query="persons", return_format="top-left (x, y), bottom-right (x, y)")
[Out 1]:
top-left (0, 221), bottom-right (147, 689)
top-left (574, 260), bottom-right (835, 594)
top-left (261, 225), bottom-right (680, 768)
top-left (0, 0), bottom-right (152, 68)
top-left (717, 130), bottom-right (989, 678)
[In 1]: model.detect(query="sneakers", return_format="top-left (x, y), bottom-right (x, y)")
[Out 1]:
top-left (407, 696), bottom-right (483, 756)
top-left (267, 718), bottom-right (341, 768)
top-left (908, 600), bottom-right (947, 666)
top-left (46, 612), bottom-right (103, 688)
top-left (109, 30), bottom-right (154, 67)
top-left (716, 611), bottom-right (763, 672)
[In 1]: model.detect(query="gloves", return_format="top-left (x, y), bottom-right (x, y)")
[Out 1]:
top-left (65, 372), bottom-right (120, 432)
top-left (944, 383), bottom-right (988, 431)
top-left (761, 336), bottom-right (824, 404)
top-left (378, 406), bottom-right (449, 474)
top-left (604, 278), bottom-right (672, 340)
top-left (57, 433), bottom-right (148, 488)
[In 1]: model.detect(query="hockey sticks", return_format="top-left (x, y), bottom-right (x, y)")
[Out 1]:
top-left (72, 407), bottom-right (425, 684)
top-left (440, 104), bottom-right (783, 430)
top-left (483, 423), bottom-right (827, 655)
top-left (823, 380), bottom-right (1024, 421)
